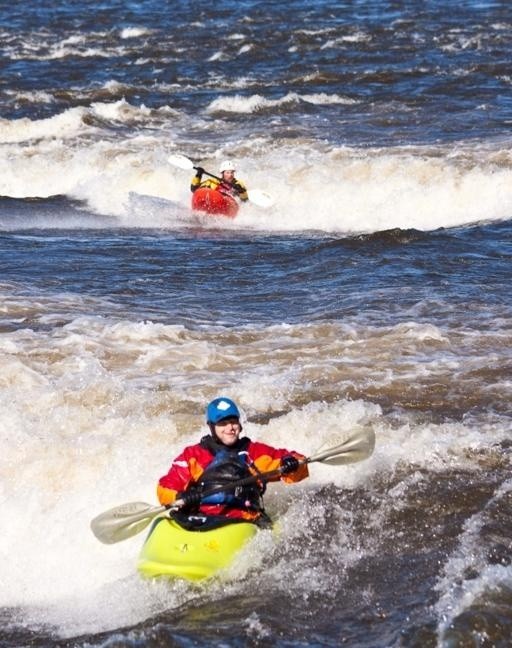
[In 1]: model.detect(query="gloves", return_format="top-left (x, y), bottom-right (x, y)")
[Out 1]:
top-left (281, 457), bottom-right (298, 473)
top-left (182, 490), bottom-right (201, 505)
top-left (196, 167), bottom-right (204, 177)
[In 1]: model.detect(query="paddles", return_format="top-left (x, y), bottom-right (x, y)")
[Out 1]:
top-left (167, 153), bottom-right (274, 207)
top-left (91, 424), bottom-right (376, 545)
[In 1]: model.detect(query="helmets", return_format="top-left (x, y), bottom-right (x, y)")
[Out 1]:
top-left (207, 397), bottom-right (240, 424)
top-left (220, 160), bottom-right (236, 173)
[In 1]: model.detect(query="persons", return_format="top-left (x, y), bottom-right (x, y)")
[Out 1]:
top-left (154, 394), bottom-right (311, 531)
top-left (188, 158), bottom-right (250, 203)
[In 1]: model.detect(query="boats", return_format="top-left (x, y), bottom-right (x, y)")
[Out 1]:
top-left (133, 500), bottom-right (262, 586)
top-left (192, 187), bottom-right (238, 218)
